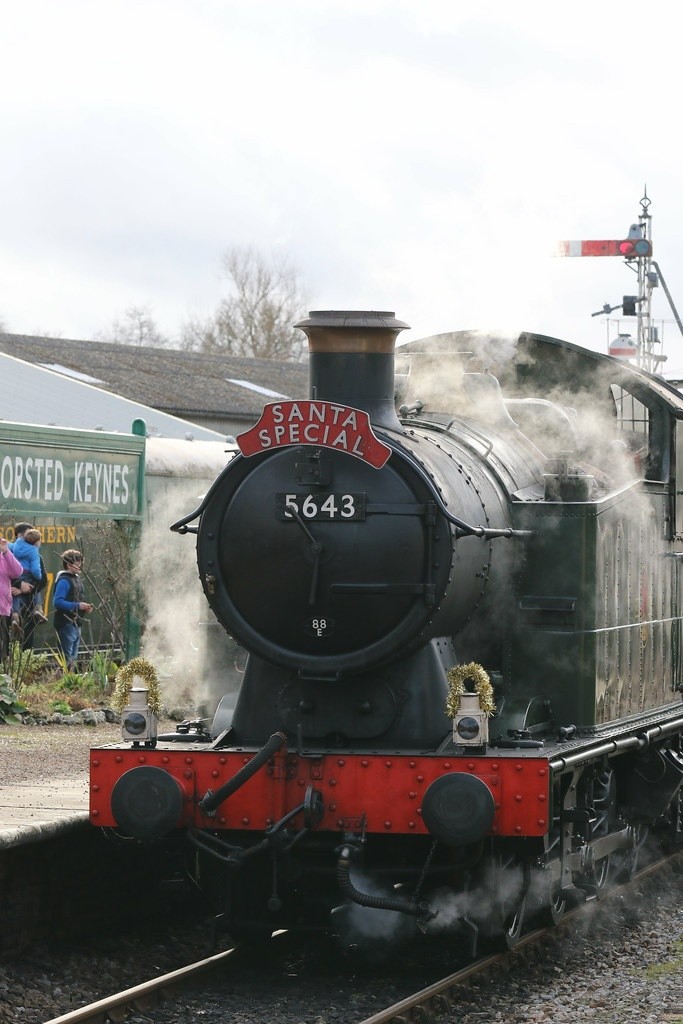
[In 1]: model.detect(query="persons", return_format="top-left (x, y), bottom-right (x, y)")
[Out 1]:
top-left (0, 523), bottom-right (48, 660)
top-left (54, 550), bottom-right (94, 665)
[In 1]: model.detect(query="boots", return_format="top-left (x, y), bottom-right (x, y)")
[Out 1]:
top-left (11, 611), bottom-right (23, 633)
top-left (34, 603), bottom-right (48, 625)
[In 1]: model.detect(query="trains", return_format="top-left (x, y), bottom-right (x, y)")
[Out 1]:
top-left (91, 310), bottom-right (682, 977)
